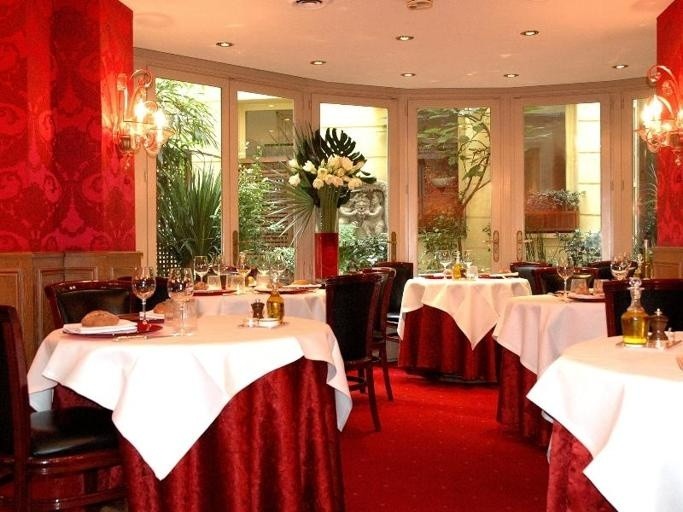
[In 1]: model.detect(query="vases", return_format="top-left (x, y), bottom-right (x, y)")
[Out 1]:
top-left (314, 205), bottom-right (339, 282)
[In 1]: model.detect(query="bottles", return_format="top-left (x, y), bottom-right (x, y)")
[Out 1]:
top-left (266, 278), bottom-right (285, 319)
top-left (647, 308), bottom-right (670, 348)
top-left (622, 277), bottom-right (650, 343)
top-left (641, 240), bottom-right (654, 278)
top-left (251, 299), bottom-right (265, 318)
top-left (453, 251), bottom-right (462, 279)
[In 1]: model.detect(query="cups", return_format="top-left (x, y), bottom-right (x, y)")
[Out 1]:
top-left (593, 279), bottom-right (608, 295)
top-left (570, 279), bottom-right (586, 293)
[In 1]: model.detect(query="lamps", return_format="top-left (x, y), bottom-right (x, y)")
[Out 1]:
top-left (636, 64), bottom-right (683, 167)
top-left (115, 69), bottom-right (164, 169)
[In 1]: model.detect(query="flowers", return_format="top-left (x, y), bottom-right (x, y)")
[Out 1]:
top-left (260, 118), bottom-right (376, 263)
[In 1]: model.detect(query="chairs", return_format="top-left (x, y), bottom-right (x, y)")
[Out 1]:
top-left (510, 261), bottom-right (638, 294)
top-left (0, 306), bottom-right (127, 512)
top-left (603, 279), bottom-right (683, 336)
top-left (351, 267), bottom-right (396, 400)
top-left (44, 280), bottom-right (132, 329)
top-left (321, 272), bottom-right (388, 432)
top-left (118, 276), bottom-right (172, 313)
top-left (374, 262), bottom-right (413, 344)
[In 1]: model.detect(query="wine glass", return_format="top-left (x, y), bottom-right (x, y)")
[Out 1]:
top-left (556, 255), bottom-right (574, 300)
top-left (211, 256), bottom-right (227, 286)
top-left (610, 260), bottom-right (629, 280)
top-left (255, 255), bottom-right (270, 274)
top-left (131, 266), bottom-right (158, 325)
top-left (270, 252), bottom-right (289, 281)
top-left (437, 249), bottom-right (452, 279)
top-left (193, 256), bottom-right (210, 283)
top-left (460, 250), bottom-right (475, 281)
top-left (235, 264), bottom-right (251, 276)
top-left (167, 268), bottom-right (195, 336)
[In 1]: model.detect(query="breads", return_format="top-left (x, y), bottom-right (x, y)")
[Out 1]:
top-left (81, 309), bottom-right (120, 327)
top-left (154, 299), bottom-right (175, 314)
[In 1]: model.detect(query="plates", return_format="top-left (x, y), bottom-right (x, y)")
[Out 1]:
top-left (254, 287), bottom-right (310, 293)
top-left (62, 326), bottom-right (163, 338)
top-left (193, 290), bottom-right (237, 295)
top-left (568, 294), bottom-right (606, 302)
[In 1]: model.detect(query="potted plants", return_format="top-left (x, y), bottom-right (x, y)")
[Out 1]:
top-left (525, 189), bottom-right (581, 232)
